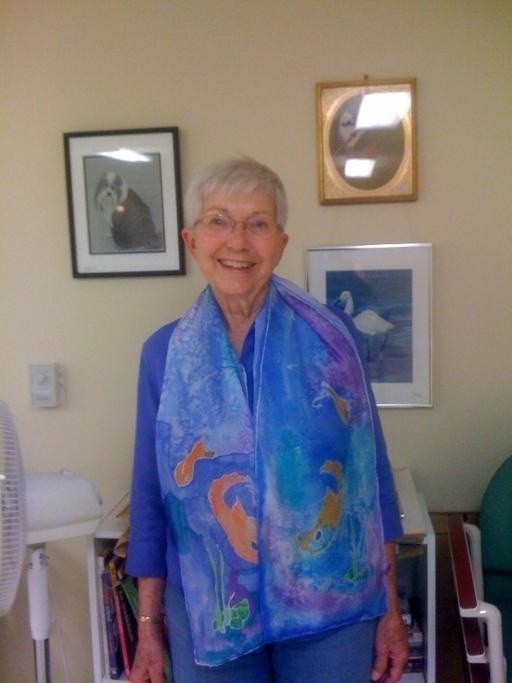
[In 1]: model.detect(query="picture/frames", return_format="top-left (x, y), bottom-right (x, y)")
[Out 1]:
top-left (61, 126), bottom-right (186, 279)
top-left (316, 76), bottom-right (418, 206)
top-left (304, 240), bottom-right (432, 411)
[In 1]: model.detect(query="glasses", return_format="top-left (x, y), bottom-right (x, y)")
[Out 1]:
top-left (196, 212), bottom-right (282, 239)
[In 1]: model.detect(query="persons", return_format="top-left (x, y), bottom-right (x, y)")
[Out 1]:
top-left (122, 154), bottom-right (410, 683)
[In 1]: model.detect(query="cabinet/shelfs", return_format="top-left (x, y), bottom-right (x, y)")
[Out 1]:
top-left (86, 485), bottom-right (436, 682)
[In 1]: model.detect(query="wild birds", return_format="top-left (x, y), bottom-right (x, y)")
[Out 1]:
top-left (332, 289), bottom-right (399, 366)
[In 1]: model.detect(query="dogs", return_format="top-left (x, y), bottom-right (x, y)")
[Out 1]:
top-left (95, 172), bottom-right (162, 250)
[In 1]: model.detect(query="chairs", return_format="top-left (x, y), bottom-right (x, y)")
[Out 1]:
top-left (445, 509), bottom-right (507, 683)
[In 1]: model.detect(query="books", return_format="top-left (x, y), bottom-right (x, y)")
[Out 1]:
top-left (396, 595), bottom-right (426, 676)
top-left (98, 544), bottom-right (172, 682)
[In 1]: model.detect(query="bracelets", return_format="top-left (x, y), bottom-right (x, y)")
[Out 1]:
top-left (136, 615), bottom-right (161, 624)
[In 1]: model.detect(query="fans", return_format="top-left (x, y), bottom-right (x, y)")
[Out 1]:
top-left (0, 401), bottom-right (103, 683)
